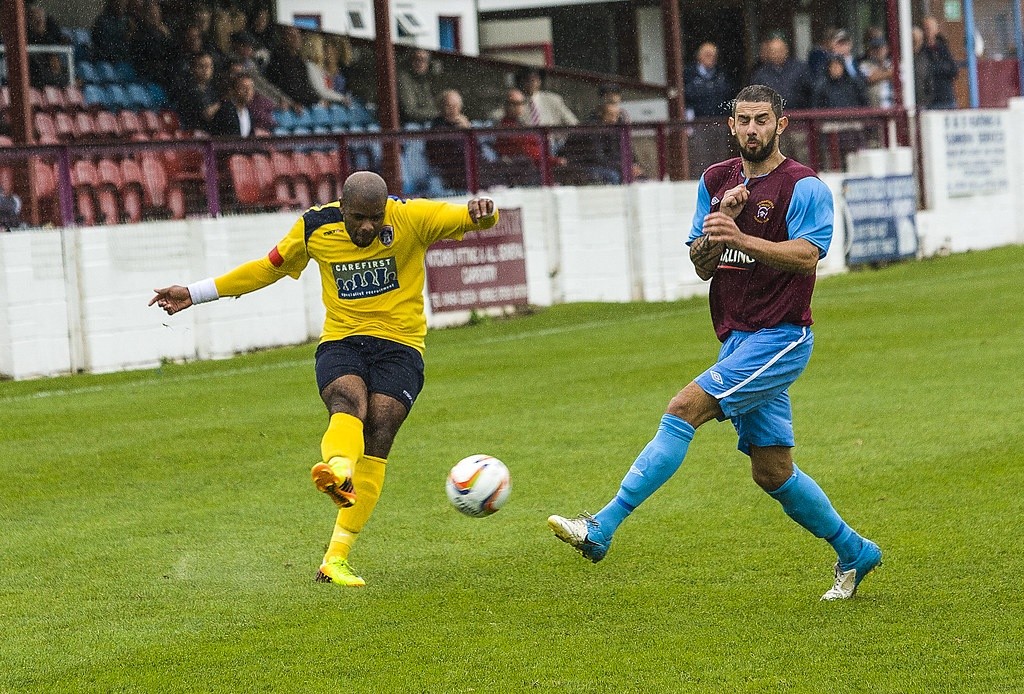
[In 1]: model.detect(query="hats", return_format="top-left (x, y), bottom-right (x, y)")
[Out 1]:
top-left (834, 30), bottom-right (852, 43)
top-left (869, 39), bottom-right (886, 48)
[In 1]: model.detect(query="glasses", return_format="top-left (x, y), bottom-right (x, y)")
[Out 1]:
top-left (506, 99), bottom-right (528, 106)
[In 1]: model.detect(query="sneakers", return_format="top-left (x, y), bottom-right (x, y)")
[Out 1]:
top-left (311, 461), bottom-right (357, 508)
top-left (548, 509), bottom-right (612, 564)
top-left (316, 556), bottom-right (366, 587)
top-left (819, 538), bottom-right (882, 599)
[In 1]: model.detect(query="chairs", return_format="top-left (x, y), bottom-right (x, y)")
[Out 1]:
top-left (0, 25), bottom-right (600, 233)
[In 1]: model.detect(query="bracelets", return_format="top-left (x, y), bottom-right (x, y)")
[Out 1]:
top-left (187, 276), bottom-right (220, 305)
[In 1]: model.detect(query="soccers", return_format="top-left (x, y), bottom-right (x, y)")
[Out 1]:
top-left (444, 454), bottom-right (511, 519)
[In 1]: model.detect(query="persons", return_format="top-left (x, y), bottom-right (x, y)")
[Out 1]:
top-left (551, 86), bottom-right (884, 604)
top-left (147, 171), bottom-right (499, 586)
top-left (0, 0), bottom-right (959, 189)
